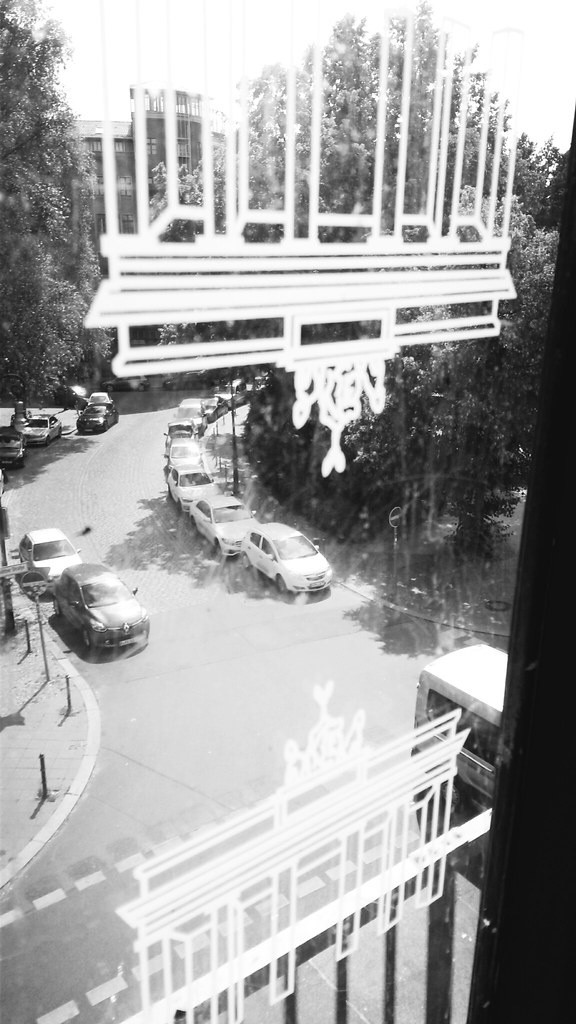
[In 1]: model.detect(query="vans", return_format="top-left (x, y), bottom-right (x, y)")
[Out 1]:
top-left (416, 642), bottom-right (525, 808)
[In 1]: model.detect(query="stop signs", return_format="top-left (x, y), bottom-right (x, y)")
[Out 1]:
top-left (20, 571), bottom-right (48, 596)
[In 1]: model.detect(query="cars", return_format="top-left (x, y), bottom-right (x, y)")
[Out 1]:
top-left (86, 392), bottom-right (114, 405)
top-left (21, 413), bottom-right (63, 447)
top-left (0, 431), bottom-right (26, 470)
top-left (54, 563), bottom-right (150, 655)
top-left (18, 527), bottom-right (83, 593)
top-left (53, 384), bottom-right (89, 410)
top-left (77, 403), bottom-right (120, 434)
top-left (189, 494), bottom-right (263, 562)
top-left (169, 436), bottom-right (204, 474)
top-left (167, 464), bottom-right (219, 515)
top-left (241, 522), bottom-right (333, 596)
top-left (100, 364), bottom-right (273, 457)
